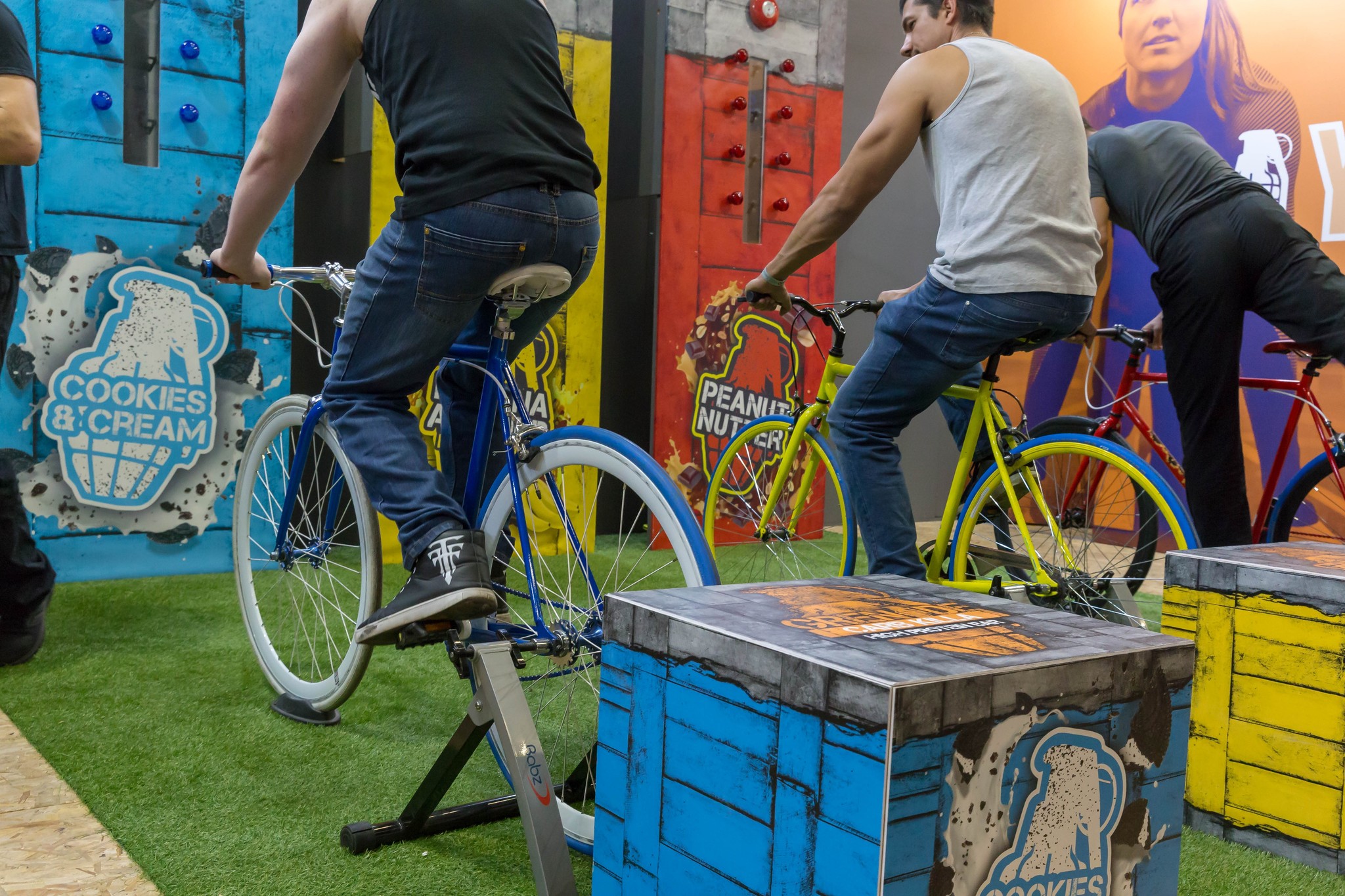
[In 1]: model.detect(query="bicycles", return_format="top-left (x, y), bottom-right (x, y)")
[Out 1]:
top-left (700, 289), bottom-right (1204, 632)
top-left (199, 258), bottom-right (724, 862)
top-left (992, 323), bottom-right (1345, 622)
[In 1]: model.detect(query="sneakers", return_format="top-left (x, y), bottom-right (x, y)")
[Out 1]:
top-left (956, 446), bottom-right (1040, 517)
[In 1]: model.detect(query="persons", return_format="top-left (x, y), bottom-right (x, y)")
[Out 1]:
top-left (210, 0), bottom-right (601, 644)
top-left (744, 0), bottom-right (1103, 583)
top-left (0, 0), bottom-right (57, 664)
top-left (1017, 0), bottom-right (1317, 538)
top-left (1086, 119), bottom-right (1345, 549)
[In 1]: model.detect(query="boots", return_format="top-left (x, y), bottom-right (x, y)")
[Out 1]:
top-left (356, 528), bottom-right (513, 645)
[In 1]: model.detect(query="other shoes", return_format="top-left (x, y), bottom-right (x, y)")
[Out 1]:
top-left (0, 580), bottom-right (58, 669)
